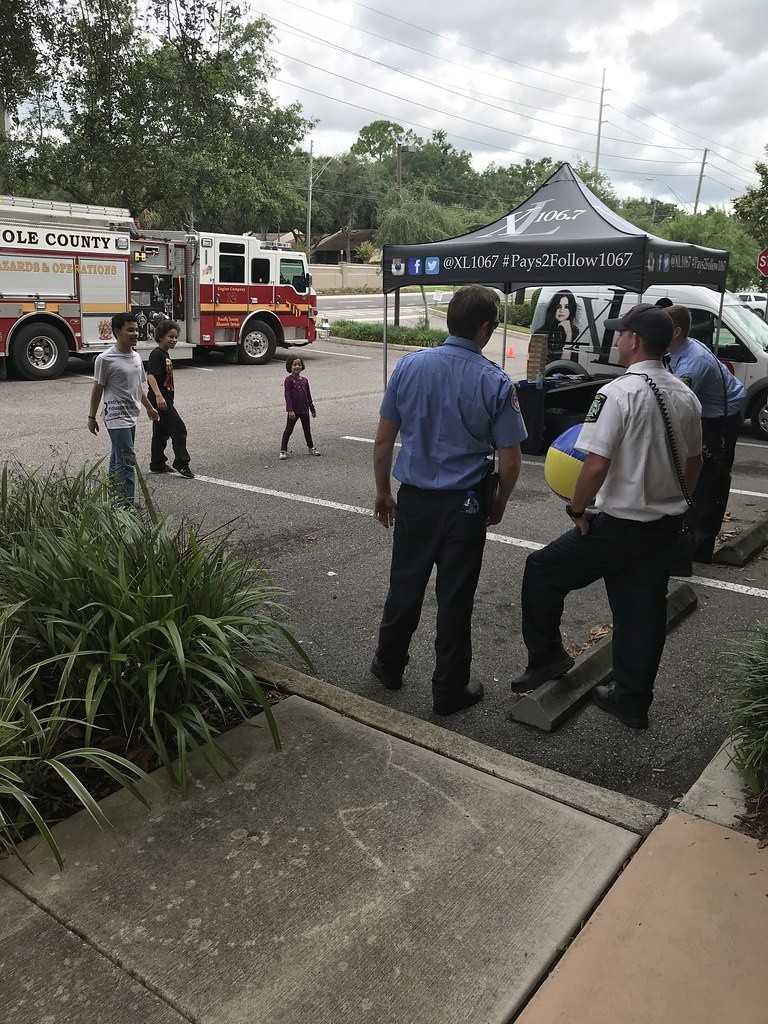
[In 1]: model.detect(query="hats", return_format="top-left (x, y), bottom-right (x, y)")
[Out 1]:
top-left (604, 303), bottom-right (673, 348)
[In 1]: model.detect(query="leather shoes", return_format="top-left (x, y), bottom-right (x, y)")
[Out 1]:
top-left (510, 651), bottom-right (575, 692)
top-left (371, 655), bottom-right (403, 690)
top-left (591, 686), bottom-right (649, 729)
top-left (434, 679), bottom-right (483, 716)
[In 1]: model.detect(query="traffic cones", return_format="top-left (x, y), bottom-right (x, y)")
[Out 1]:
top-left (506, 346), bottom-right (516, 359)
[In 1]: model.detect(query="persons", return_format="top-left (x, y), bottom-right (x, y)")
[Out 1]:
top-left (534, 290), bottom-right (579, 365)
top-left (655, 298), bottom-right (673, 369)
top-left (146, 320), bottom-right (195, 478)
top-left (88, 313), bottom-right (160, 509)
top-left (512, 303), bottom-right (702, 729)
top-left (661, 305), bottom-right (748, 564)
top-left (278, 355), bottom-right (320, 459)
top-left (370, 285), bottom-right (529, 714)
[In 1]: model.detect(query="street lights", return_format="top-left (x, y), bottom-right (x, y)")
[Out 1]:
top-left (644, 175), bottom-right (703, 243)
top-left (306, 150), bottom-right (350, 264)
top-left (393, 143), bottom-right (418, 327)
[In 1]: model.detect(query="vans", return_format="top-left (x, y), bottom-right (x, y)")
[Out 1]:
top-left (525, 286), bottom-right (767, 445)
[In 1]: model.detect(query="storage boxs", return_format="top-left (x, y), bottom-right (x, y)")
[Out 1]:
top-left (527, 334), bottom-right (549, 381)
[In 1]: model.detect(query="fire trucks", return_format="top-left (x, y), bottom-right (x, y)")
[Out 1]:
top-left (0, 194), bottom-right (332, 382)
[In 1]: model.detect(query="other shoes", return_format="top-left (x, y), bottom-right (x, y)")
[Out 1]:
top-left (279, 450), bottom-right (289, 459)
top-left (693, 547), bottom-right (712, 562)
top-left (669, 560), bottom-right (693, 576)
top-left (309, 447), bottom-right (321, 455)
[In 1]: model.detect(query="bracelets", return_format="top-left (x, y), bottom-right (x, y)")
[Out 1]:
top-left (88, 416), bottom-right (96, 420)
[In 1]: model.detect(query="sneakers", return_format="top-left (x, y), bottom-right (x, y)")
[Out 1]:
top-left (148, 464), bottom-right (174, 473)
top-left (171, 461), bottom-right (195, 479)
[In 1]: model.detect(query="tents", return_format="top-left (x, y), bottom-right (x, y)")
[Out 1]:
top-left (382, 162), bottom-right (729, 396)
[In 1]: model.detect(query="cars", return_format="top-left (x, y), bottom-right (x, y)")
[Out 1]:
top-left (714, 291), bottom-right (768, 328)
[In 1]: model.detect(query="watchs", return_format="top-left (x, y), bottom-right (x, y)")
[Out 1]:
top-left (566, 503), bottom-right (584, 519)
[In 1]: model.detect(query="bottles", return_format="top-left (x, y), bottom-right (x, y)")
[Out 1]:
top-left (536, 372), bottom-right (543, 389)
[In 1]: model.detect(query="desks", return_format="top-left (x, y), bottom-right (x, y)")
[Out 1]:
top-left (515, 375), bottom-right (617, 455)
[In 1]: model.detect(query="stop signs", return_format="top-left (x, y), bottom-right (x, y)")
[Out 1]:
top-left (756, 247), bottom-right (768, 278)
